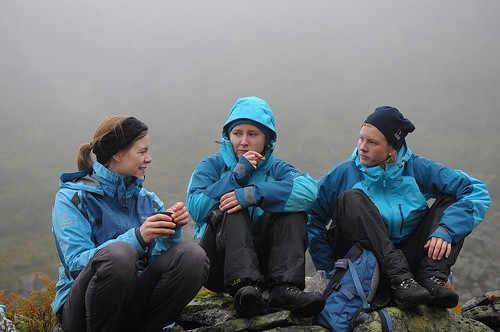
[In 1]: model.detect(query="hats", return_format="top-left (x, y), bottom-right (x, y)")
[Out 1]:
top-left (364, 106), bottom-right (415, 152)
top-left (228, 119), bottom-right (271, 141)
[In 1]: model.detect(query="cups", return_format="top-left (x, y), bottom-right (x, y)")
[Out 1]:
top-left (151, 211), bottom-right (174, 238)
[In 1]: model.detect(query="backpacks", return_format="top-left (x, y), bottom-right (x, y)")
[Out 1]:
top-left (317, 241), bottom-right (395, 332)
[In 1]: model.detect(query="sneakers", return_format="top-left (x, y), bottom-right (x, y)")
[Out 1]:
top-left (391, 277), bottom-right (431, 309)
top-left (233, 285), bottom-right (266, 318)
top-left (418, 275), bottom-right (459, 311)
top-left (266, 284), bottom-right (326, 318)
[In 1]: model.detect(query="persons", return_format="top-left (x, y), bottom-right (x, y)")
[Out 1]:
top-left (308, 105), bottom-right (492, 310)
top-left (185, 96), bottom-right (327, 316)
top-left (47, 114), bottom-right (210, 332)
